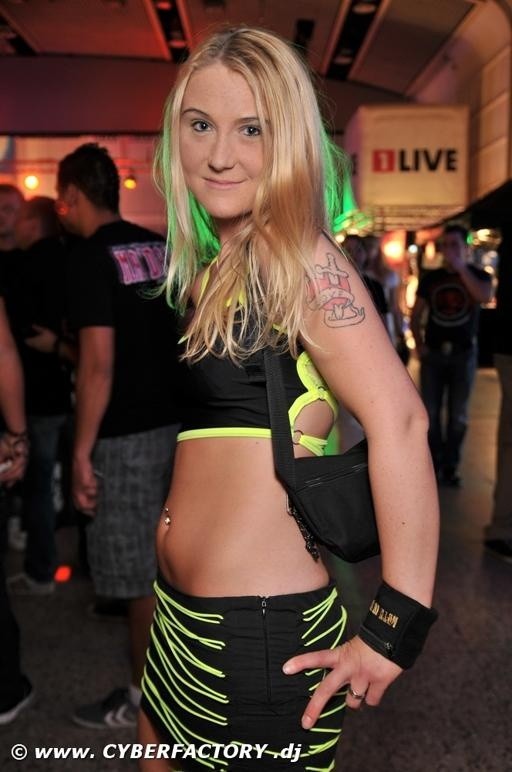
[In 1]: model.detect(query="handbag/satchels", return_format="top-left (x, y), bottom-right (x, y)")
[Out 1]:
top-left (287, 437), bottom-right (382, 564)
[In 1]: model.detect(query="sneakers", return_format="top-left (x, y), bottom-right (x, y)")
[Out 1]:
top-left (69, 687), bottom-right (137, 730)
top-left (0, 675), bottom-right (36, 726)
top-left (438, 471), bottom-right (465, 483)
top-left (481, 538), bottom-right (512, 564)
top-left (85, 599), bottom-right (131, 623)
top-left (22, 566), bottom-right (56, 595)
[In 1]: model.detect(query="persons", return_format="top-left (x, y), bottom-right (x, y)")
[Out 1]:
top-left (472, 219), bottom-right (511, 556)
top-left (366, 238), bottom-right (406, 350)
top-left (129, 23), bottom-right (446, 771)
top-left (341, 234), bottom-right (390, 329)
top-left (411, 224), bottom-right (493, 489)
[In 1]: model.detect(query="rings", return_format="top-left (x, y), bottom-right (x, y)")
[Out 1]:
top-left (347, 688), bottom-right (365, 700)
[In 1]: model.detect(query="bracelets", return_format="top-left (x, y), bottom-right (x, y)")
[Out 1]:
top-left (358, 580), bottom-right (440, 670)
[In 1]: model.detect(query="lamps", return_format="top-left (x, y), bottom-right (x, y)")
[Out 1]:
top-left (0, 156), bottom-right (155, 190)
